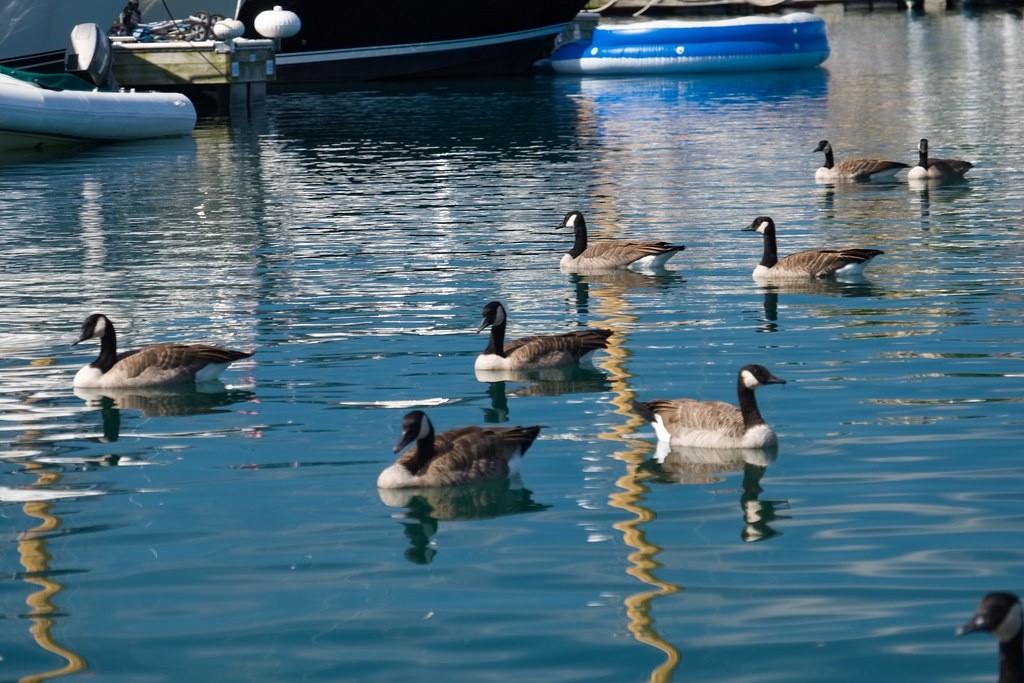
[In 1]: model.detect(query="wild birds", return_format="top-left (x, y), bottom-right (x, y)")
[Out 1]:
top-left (474, 301), bottom-right (614, 371)
top-left (376, 410), bottom-right (552, 488)
top-left (955, 593), bottom-right (1024, 683)
top-left (740, 216), bottom-right (885, 279)
top-left (379, 472), bottom-right (554, 566)
top-left (555, 211), bottom-right (685, 270)
top-left (558, 266), bottom-right (688, 327)
top-left (908, 138), bottom-right (975, 179)
top-left (628, 364), bottom-right (786, 449)
top-left (812, 140), bottom-right (912, 179)
top-left (70, 314), bottom-right (257, 387)
top-left (73, 379), bottom-right (256, 443)
top-left (639, 449), bottom-right (793, 541)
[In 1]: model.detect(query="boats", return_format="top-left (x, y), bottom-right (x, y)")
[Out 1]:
top-left (0, 0), bottom-right (592, 143)
